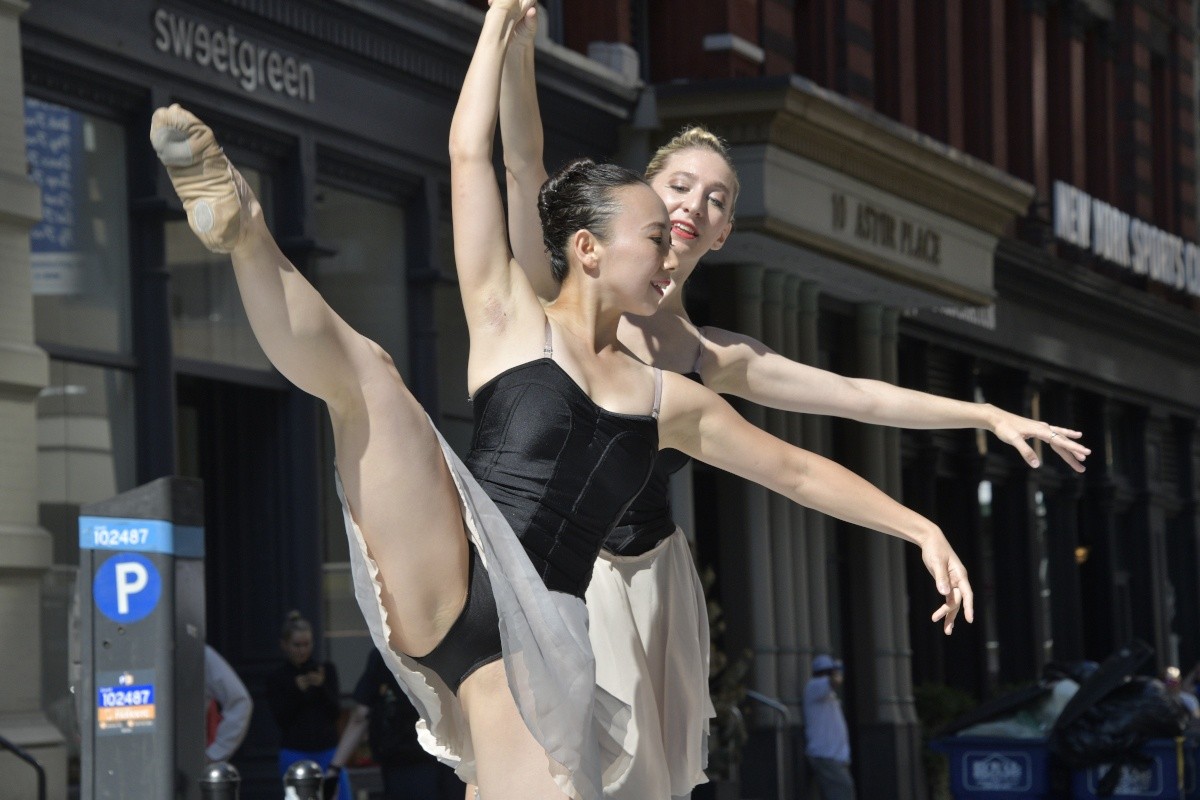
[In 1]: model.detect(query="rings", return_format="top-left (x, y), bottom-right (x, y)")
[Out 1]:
top-left (1049, 432), bottom-right (1058, 444)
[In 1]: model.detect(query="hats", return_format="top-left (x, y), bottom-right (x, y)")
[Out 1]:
top-left (811, 655), bottom-right (840, 672)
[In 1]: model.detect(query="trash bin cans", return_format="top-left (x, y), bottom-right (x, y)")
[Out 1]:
top-left (925, 644), bottom-right (1200, 800)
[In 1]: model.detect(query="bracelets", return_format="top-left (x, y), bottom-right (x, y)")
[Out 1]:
top-left (328, 762), bottom-right (342, 774)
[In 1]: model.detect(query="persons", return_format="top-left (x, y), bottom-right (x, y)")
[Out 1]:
top-left (803, 652), bottom-right (1200, 800)
top-left (202, 609), bottom-right (468, 800)
top-left (146, 1), bottom-right (973, 800)
top-left (500, 9), bottom-right (1092, 800)
